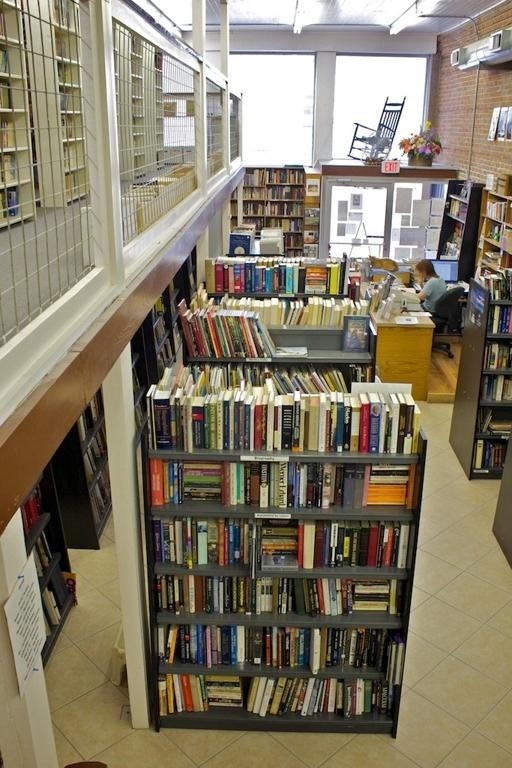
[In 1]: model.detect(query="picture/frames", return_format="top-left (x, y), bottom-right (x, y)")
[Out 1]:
top-left (487, 106), bottom-right (511, 142)
top-left (343, 315), bottom-right (370, 353)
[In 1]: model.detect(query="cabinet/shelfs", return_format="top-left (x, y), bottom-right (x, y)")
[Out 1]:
top-left (203, 253), bottom-right (349, 298)
top-left (491, 427), bottom-right (512, 568)
top-left (0, 0), bottom-right (164, 229)
top-left (475, 188), bottom-right (512, 276)
top-left (231, 166), bottom-right (265, 240)
top-left (435, 180), bottom-right (486, 283)
top-left (183, 326), bottom-right (375, 382)
top-left (266, 165), bottom-right (303, 256)
top-left (448, 278), bottom-right (511, 481)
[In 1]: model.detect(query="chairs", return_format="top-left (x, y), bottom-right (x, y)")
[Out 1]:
top-left (347, 96), bottom-right (406, 161)
top-left (431, 287), bottom-right (465, 359)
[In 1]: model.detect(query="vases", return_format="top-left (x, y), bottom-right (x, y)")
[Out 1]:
top-left (408, 156), bottom-right (432, 167)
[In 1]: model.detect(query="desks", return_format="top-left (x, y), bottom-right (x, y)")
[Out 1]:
top-left (369, 310), bottom-right (436, 401)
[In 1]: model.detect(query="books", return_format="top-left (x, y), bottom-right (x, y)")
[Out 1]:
top-left (145, 365), bottom-right (423, 719)
top-left (449, 199), bottom-right (469, 221)
top-left (472, 268), bottom-right (512, 475)
top-left (78, 392), bottom-right (111, 530)
top-left (157, 255), bottom-right (396, 359)
top-left (442, 222), bottom-right (465, 258)
top-left (230, 167), bottom-right (305, 256)
top-left (488, 199), bottom-right (512, 222)
top-left (21, 482), bottom-right (67, 639)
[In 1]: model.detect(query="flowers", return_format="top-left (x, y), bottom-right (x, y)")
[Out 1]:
top-left (398, 121), bottom-right (442, 158)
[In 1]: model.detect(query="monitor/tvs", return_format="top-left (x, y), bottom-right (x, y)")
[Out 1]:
top-left (424, 258), bottom-right (460, 282)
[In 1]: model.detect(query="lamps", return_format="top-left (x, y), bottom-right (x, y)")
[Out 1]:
top-left (388, 0), bottom-right (423, 35)
top-left (293, 0), bottom-right (306, 34)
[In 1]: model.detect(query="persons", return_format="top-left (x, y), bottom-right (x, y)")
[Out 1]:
top-left (415, 258), bottom-right (448, 313)
